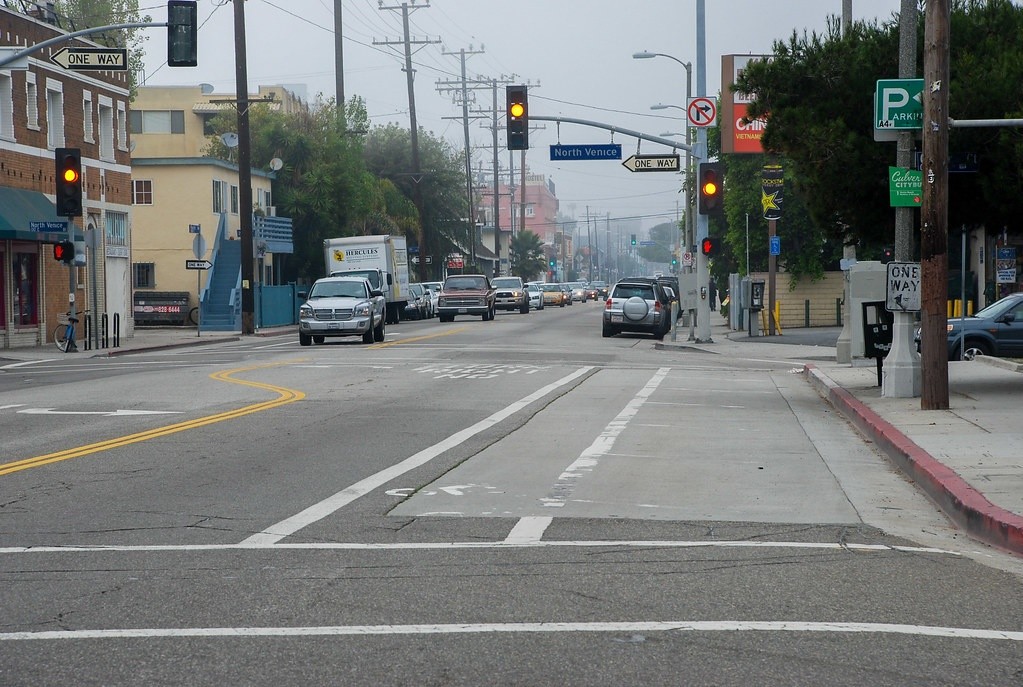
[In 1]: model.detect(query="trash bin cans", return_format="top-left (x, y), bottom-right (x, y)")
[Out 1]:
top-left (861, 301), bottom-right (894, 386)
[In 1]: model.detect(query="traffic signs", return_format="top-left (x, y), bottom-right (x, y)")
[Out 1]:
top-left (411, 256), bottom-right (432, 265)
top-left (185, 260), bottom-right (213, 270)
top-left (49, 47), bottom-right (128, 72)
top-left (885, 262), bottom-right (922, 310)
top-left (622, 154), bottom-right (680, 172)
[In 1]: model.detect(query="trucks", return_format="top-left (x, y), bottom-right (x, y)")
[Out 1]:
top-left (323, 234), bottom-right (409, 324)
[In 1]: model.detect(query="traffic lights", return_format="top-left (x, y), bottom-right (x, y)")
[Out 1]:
top-left (54, 148), bottom-right (83, 217)
top-left (672, 254), bottom-right (676, 265)
top-left (506, 85), bottom-right (529, 150)
top-left (54, 310), bottom-right (91, 353)
top-left (54, 241), bottom-right (75, 261)
top-left (630, 234), bottom-right (636, 246)
top-left (549, 256), bottom-right (556, 266)
top-left (699, 163), bottom-right (724, 215)
top-left (702, 237), bottom-right (720, 257)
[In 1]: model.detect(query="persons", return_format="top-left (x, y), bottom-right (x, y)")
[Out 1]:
top-left (709, 278), bottom-right (717, 311)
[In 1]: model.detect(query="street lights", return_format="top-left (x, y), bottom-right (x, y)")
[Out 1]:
top-left (632, 50), bottom-right (694, 341)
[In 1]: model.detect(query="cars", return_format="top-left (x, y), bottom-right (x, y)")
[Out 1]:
top-left (437, 273), bottom-right (609, 323)
top-left (297, 276), bottom-right (386, 346)
top-left (915, 292), bottom-right (1023, 361)
top-left (405, 282), bottom-right (444, 319)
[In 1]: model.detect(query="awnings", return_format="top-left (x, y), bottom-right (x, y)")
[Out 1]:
top-left (0, 184), bottom-right (85, 241)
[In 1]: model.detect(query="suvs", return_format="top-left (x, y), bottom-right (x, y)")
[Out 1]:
top-left (602, 271), bottom-right (680, 340)
